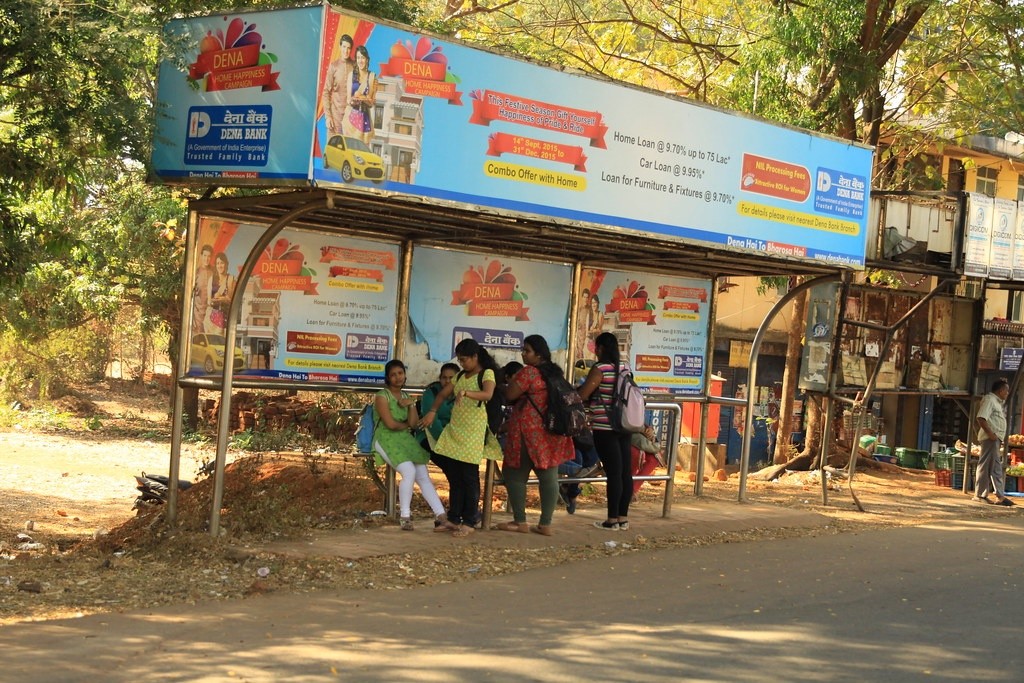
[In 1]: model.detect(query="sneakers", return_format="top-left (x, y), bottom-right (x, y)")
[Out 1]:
top-left (619, 521), bottom-right (629, 531)
top-left (593, 520), bottom-right (619, 530)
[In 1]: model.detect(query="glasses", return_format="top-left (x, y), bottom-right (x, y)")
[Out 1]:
top-left (457, 357), bottom-right (469, 363)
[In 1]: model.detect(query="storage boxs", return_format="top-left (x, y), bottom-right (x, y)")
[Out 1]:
top-left (837, 355), bottom-right (868, 388)
top-left (932, 447), bottom-right (1023, 493)
top-left (844, 412), bottom-right (877, 448)
top-left (872, 362), bottom-right (895, 389)
top-left (894, 447), bottom-right (929, 470)
top-left (906, 360), bottom-right (941, 388)
top-left (878, 445), bottom-right (891, 454)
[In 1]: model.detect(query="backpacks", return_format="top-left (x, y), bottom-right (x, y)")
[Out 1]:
top-left (353, 390), bottom-right (408, 453)
top-left (595, 360), bottom-right (645, 432)
top-left (457, 367), bottom-right (504, 436)
top-left (523, 361), bottom-right (586, 436)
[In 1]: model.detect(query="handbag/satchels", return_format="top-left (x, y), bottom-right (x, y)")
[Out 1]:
top-left (586, 338), bottom-right (596, 355)
top-left (211, 309), bottom-right (227, 329)
top-left (631, 432), bottom-right (661, 454)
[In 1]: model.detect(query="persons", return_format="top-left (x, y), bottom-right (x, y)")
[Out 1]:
top-left (414, 333), bottom-right (664, 537)
top-left (975, 380), bottom-right (1016, 506)
top-left (575, 287), bottom-right (605, 360)
top-left (321, 34), bottom-right (377, 147)
top-left (192, 246), bottom-right (235, 337)
top-left (370, 359), bottom-right (461, 531)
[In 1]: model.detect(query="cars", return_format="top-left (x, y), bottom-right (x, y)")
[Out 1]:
top-left (190, 332), bottom-right (244, 374)
top-left (573, 360), bottom-right (598, 384)
top-left (322, 135), bottom-right (387, 185)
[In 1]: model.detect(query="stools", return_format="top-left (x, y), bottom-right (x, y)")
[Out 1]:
top-left (938, 444), bottom-right (946, 452)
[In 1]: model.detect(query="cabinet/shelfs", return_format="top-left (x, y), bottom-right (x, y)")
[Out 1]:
top-left (931, 442), bottom-right (939, 460)
top-left (932, 407), bottom-right (967, 442)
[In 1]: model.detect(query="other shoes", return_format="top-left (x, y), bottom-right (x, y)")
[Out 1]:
top-left (399, 517), bottom-right (413, 531)
top-left (433, 519), bottom-right (460, 532)
top-left (453, 522), bottom-right (475, 533)
top-left (576, 461), bottom-right (598, 478)
top-left (558, 485), bottom-right (571, 507)
top-left (566, 496), bottom-right (576, 513)
top-left (473, 521), bottom-right (498, 529)
top-left (530, 524), bottom-right (551, 535)
top-left (497, 520), bottom-right (527, 533)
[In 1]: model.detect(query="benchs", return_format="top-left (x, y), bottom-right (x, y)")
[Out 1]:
top-left (339, 408), bottom-right (565, 518)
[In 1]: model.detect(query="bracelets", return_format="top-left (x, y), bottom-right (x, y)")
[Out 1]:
top-left (410, 403), bottom-right (416, 408)
top-left (429, 410), bottom-right (437, 413)
top-left (463, 391), bottom-right (467, 396)
